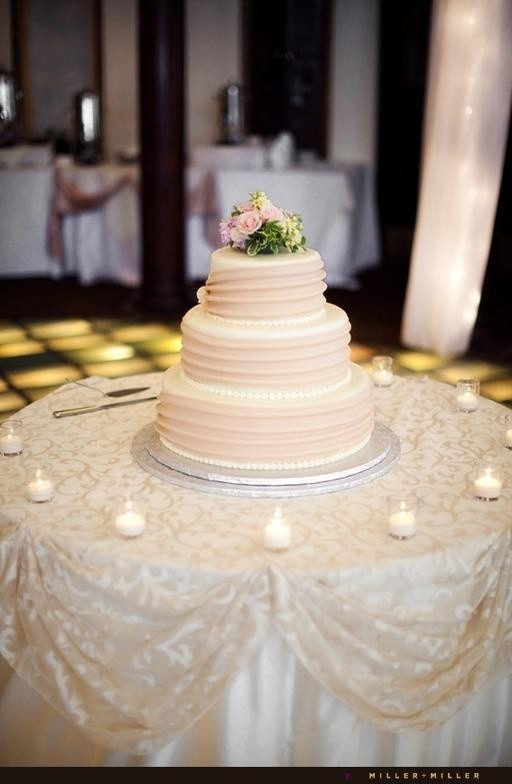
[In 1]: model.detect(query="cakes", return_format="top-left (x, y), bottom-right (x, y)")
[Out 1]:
top-left (153, 245), bottom-right (376, 474)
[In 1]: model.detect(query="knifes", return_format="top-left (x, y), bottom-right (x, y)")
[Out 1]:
top-left (53, 397), bottom-right (157, 418)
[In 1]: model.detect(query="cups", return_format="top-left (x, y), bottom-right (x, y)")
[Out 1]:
top-left (505, 415), bottom-right (512, 447)
top-left (259, 511), bottom-right (293, 553)
top-left (474, 457), bottom-right (505, 501)
top-left (0, 420), bottom-right (23, 457)
top-left (455, 379), bottom-right (481, 414)
top-left (114, 502), bottom-right (147, 540)
top-left (21, 462), bottom-right (55, 503)
top-left (373, 356), bottom-right (393, 388)
top-left (389, 494), bottom-right (418, 539)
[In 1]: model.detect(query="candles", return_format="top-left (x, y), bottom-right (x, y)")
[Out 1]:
top-left (371, 350), bottom-right (489, 416)
top-left (4, 417), bottom-right (512, 555)
